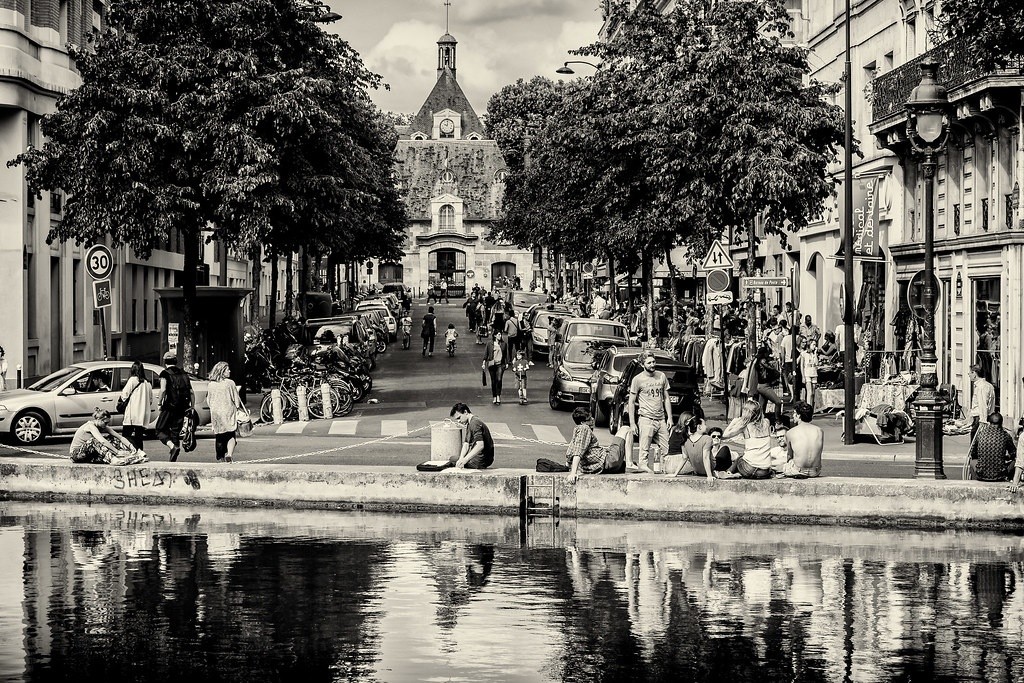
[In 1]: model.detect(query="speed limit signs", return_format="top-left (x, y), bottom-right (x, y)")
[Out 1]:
top-left (85, 244), bottom-right (114, 280)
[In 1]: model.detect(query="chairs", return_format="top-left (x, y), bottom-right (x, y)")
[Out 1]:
top-left (937, 383), bottom-right (967, 420)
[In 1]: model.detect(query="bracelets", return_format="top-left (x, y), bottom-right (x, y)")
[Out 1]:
top-left (129, 443), bottom-right (131, 448)
top-left (435, 331), bottom-right (437, 332)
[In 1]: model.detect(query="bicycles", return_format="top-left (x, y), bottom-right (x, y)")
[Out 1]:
top-left (244, 321), bottom-right (373, 424)
top-left (399, 323), bottom-right (413, 350)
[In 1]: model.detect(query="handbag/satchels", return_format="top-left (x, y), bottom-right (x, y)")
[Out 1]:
top-left (237, 419), bottom-right (253, 437)
top-left (483, 369), bottom-right (487, 386)
top-left (535, 458), bottom-right (569, 472)
top-left (116, 397), bottom-right (130, 413)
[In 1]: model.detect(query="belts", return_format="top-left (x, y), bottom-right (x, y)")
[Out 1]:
top-left (641, 415), bottom-right (662, 421)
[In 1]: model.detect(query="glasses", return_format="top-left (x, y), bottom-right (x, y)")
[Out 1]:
top-left (712, 435), bottom-right (722, 439)
top-left (101, 419), bottom-right (109, 425)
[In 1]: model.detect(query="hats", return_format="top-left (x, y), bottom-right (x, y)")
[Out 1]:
top-left (164, 352), bottom-right (176, 359)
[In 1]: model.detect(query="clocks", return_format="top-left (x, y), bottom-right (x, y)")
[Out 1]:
top-left (440, 119), bottom-right (454, 133)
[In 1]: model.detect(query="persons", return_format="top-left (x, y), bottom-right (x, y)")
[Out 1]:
top-left (565, 406), bottom-right (645, 484)
top-left (206, 361), bottom-right (241, 463)
top-left (502, 275), bottom-right (873, 416)
top-left (155, 352), bottom-right (196, 462)
top-left (421, 306), bottom-right (437, 358)
top-left (427, 283), bottom-right (439, 304)
top-left (69, 406), bottom-right (136, 465)
top-left (463, 283), bottom-right (505, 332)
top-left (360, 280), bottom-right (378, 294)
top-left (437, 278), bottom-right (449, 304)
top-left (448, 402), bottom-right (494, 469)
top-left (481, 330), bottom-right (509, 406)
top-left (399, 289), bottom-right (413, 349)
top-left (116, 361), bottom-right (153, 451)
top-left (76, 372), bottom-right (111, 393)
top-left (629, 351), bottom-right (674, 474)
top-left (444, 323), bottom-right (458, 352)
top-left (968, 365), bottom-right (1024, 493)
top-left (781, 401), bottom-right (824, 478)
top-left (0, 346), bottom-right (8, 393)
top-left (664, 400), bottom-right (790, 479)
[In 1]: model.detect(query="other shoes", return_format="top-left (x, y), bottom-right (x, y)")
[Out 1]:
top-left (170, 446), bottom-right (180, 461)
top-left (428, 353), bottom-right (432, 356)
top-left (422, 347), bottom-right (426, 355)
top-left (625, 466), bottom-right (648, 473)
top-left (493, 399), bottom-right (496, 403)
top-left (497, 399), bottom-right (501, 403)
top-left (226, 456), bottom-right (232, 462)
top-left (524, 398), bottom-right (527, 403)
top-left (110, 459), bottom-right (127, 466)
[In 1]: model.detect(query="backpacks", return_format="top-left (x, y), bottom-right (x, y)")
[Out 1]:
top-left (165, 369), bottom-right (190, 406)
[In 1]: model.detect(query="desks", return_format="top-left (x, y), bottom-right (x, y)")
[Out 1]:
top-left (813, 389), bottom-right (845, 413)
top-left (857, 383), bottom-right (921, 411)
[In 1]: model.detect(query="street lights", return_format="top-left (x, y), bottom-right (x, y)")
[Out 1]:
top-left (902, 59), bottom-right (947, 479)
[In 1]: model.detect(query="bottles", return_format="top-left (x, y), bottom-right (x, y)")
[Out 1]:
top-left (653, 449), bottom-right (661, 474)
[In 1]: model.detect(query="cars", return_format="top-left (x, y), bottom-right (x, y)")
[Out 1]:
top-left (608, 357), bottom-right (704, 435)
top-left (0, 361), bottom-right (211, 446)
top-left (589, 350), bottom-right (679, 427)
top-left (303, 282), bottom-right (413, 371)
top-left (547, 335), bottom-right (630, 411)
top-left (496, 287), bottom-right (633, 373)
